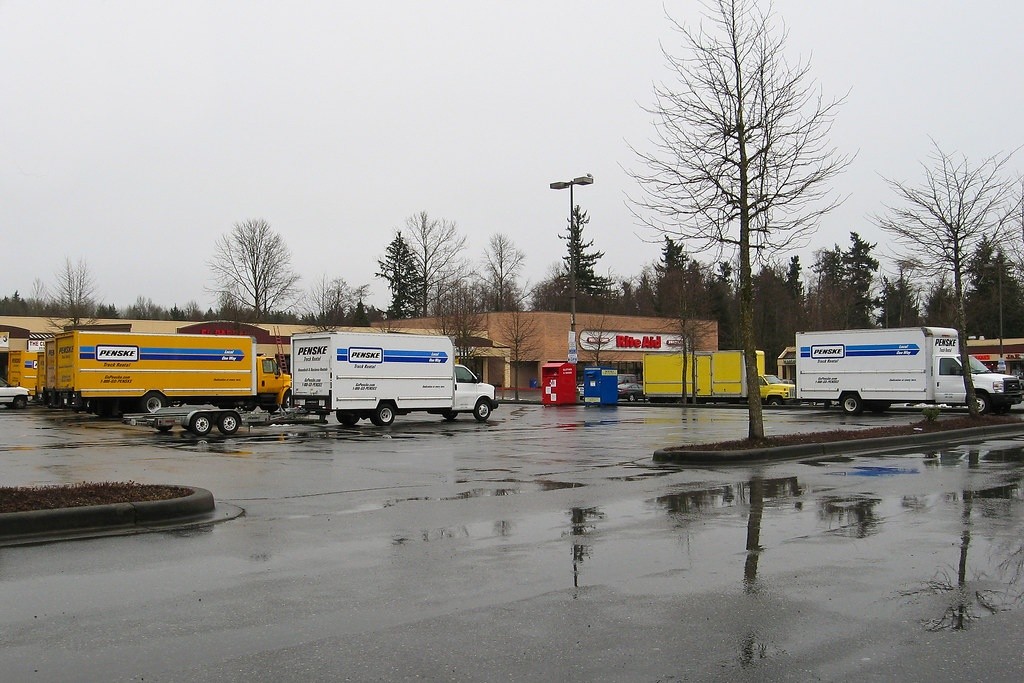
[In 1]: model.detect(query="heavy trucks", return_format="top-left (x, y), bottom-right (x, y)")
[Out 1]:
top-left (641, 349), bottom-right (801, 406)
top-left (55, 330), bottom-right (290, 415)
top-left (8, 337), bottom-right (58, 408)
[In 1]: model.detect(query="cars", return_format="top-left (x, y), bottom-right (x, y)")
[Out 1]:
top-left (618, 382), bottom-right (648, 402)
top-left (0, 377), bottom-right (34, 410)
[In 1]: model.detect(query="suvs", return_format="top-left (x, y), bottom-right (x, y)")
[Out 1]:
top-left (576, 383), bottom-right (584, 399)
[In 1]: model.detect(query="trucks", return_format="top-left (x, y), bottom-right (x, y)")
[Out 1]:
top-left (796, 326), bottom-right (1024, 414)
top-left (289, 329), bottom-right (499, 425)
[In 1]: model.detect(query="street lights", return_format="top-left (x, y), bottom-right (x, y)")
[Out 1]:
top-left (550, 172), bottom-right (594, 365)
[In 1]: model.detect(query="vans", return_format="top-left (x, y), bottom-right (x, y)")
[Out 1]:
top-left (617, 373), bottom-right (637, 384)
